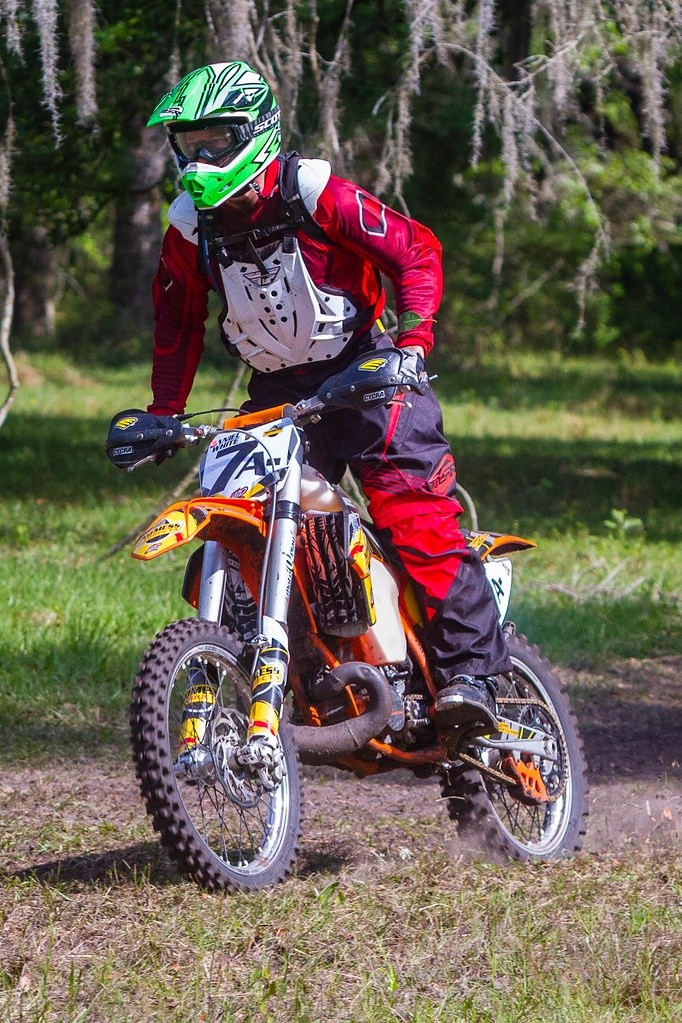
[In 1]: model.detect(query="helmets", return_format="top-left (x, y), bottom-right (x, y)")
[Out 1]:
top-left (147, 61), bottom-right (281, 210)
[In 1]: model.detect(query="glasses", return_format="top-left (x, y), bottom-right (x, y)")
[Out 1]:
top-left (168, 124), bottom-right (244, 164)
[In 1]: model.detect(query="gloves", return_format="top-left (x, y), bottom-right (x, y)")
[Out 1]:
top-left (395, 348), bottom-right (425, 395)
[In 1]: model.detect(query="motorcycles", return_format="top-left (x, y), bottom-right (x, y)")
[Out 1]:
top-left (124, 372), bottom-right (593, 901)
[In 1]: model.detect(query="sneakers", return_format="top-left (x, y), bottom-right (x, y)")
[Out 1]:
top-left (435, 674), bottom-right (500, 733)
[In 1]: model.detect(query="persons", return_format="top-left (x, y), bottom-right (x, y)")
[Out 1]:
top-left (102, 60), bottom-right (511, 736)
